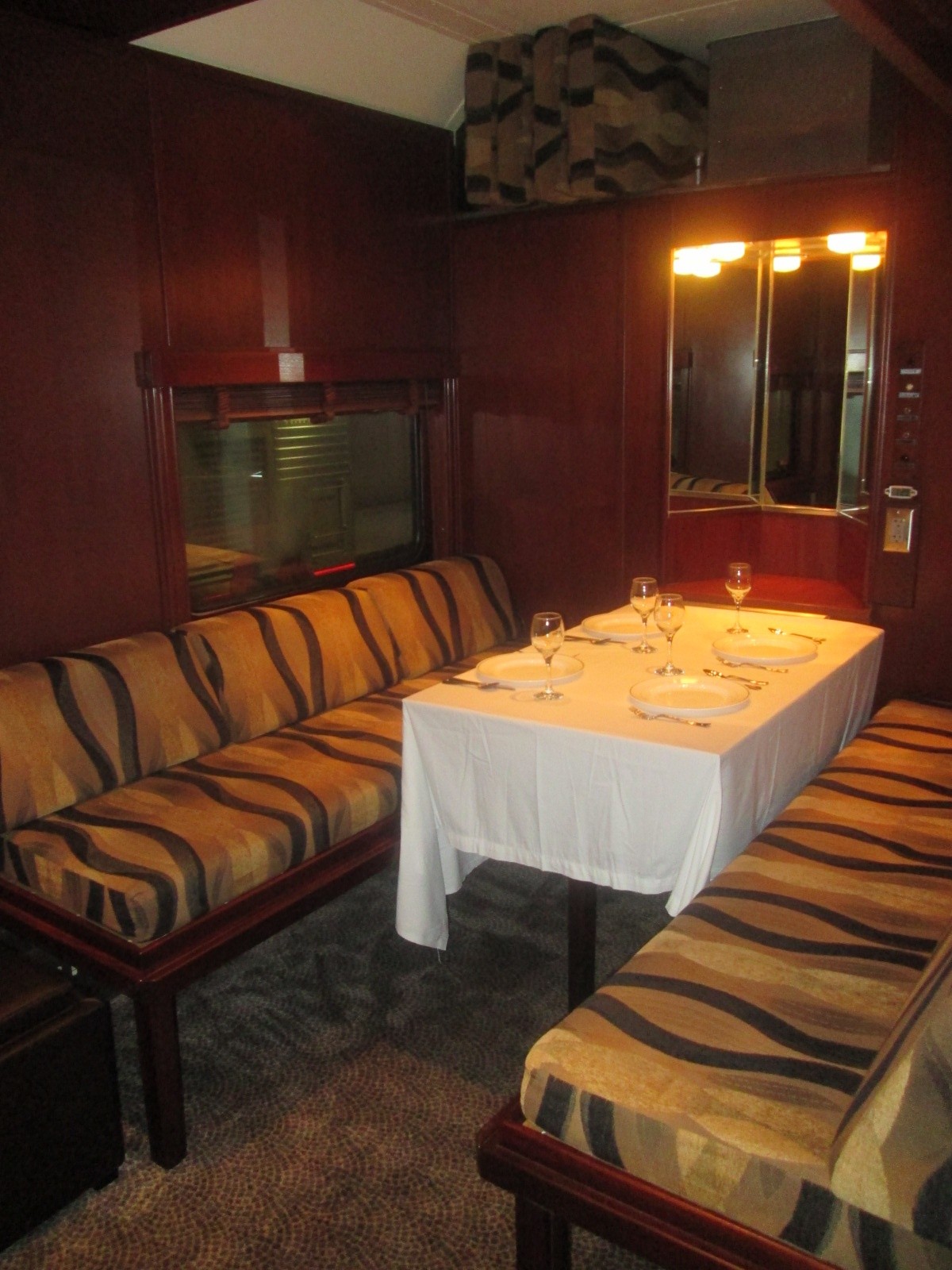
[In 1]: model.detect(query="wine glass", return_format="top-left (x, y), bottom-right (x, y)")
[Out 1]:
top-left (530, 612), bottom-right (565, 699)
top-left (654, 594), bottom-right (685, 674)
top-left (630, 578), bottom-right (661, 652)
top-left (725, 563), bottom-right (752, 632)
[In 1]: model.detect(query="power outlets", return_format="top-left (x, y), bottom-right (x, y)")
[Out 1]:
top-left (884, 505), bottom-right (914, 554)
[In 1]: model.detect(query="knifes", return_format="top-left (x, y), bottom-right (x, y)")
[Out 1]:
top-left (565, 638), bottom-right (627, 644)
top-left (447, 676), bottom-right (514, 691)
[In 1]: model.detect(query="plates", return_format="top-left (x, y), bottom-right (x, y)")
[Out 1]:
top-left (628, 674), bottom-right (751, 717)
top-left (582, 614), bottom-right (676, 641)
top-left (474, 651), bottom-right (585, 684)
top-left (712, 632), bottom-right (818, 665)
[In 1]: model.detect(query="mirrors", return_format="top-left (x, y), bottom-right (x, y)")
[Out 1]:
top-left (670, 252), bottom-right (760, 501)
top-left (664, 232), bottom-right (887, 530)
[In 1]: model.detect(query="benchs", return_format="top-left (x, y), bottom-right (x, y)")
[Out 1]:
top-left (0, 551), bottom-right (526, 1170)
top-left (469, 700), bottom-right (952, 1270)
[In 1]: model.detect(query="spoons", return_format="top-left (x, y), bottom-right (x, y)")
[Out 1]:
top-left (443, 677), bottom-right (498, 690)
top-left (768, 627), bottom-right (826, 645)
top-left (561, 635), bottom-right (611, 644)
top-left (704, 669), bottom-right (769, 686)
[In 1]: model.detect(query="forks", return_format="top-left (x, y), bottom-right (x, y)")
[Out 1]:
top-left (716, 656), bottom-right (790, 674)
top-left (629, 707), bottom-right (712, 727)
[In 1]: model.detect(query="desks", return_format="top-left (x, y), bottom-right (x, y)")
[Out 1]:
top-left (395, 603), bottom-right (887, 1016)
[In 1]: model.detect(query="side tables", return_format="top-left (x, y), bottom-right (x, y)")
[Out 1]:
top-left (1, 937), bottom-right (125, 1253)
